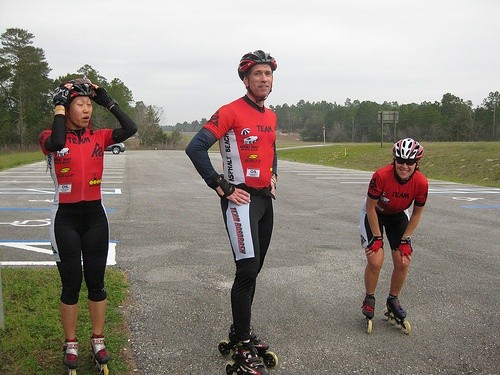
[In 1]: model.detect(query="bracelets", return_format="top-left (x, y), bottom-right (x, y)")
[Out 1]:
top-left (55, 107), bottom-right (65, 111)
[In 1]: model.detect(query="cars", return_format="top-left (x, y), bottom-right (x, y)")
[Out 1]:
top-left (104, 142), bottom-right (125, 155)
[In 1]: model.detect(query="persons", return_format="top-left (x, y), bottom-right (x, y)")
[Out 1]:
top-left (185, 50), bottom-right (278, 375)
top-left (359, 138), bottom-right (428, 335)
top-left (38, 79), bottom-right (138, 375)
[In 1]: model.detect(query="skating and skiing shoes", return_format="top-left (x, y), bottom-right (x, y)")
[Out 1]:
top-left (384, 297), bottom-right (411, 335)
top-left (218, 324), bottom-right (278, 368)
top-left (361, 295), bottom-right (375, 334)
top-left (225, 337), bottom-right (270, 375)
top-left (61, 336), bottom-right (80, 375)
top-left (90, 331), bottom-right (110, 375)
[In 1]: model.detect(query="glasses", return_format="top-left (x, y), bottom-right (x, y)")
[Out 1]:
top-left (396, 156), bottom-right (418, 166)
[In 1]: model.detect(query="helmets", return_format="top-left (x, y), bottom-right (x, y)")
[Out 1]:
top-left (59, 79), bottom-right (97, 106)
top-left (238, 50), bottom-right (277, 81)
top-left (392, 137), bottom-right (424, 161)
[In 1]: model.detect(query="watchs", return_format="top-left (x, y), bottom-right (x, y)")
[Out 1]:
top-left (406, 236), bottom-right (411, 241)
top-left (107, 98), bottom-right (118, 109)
